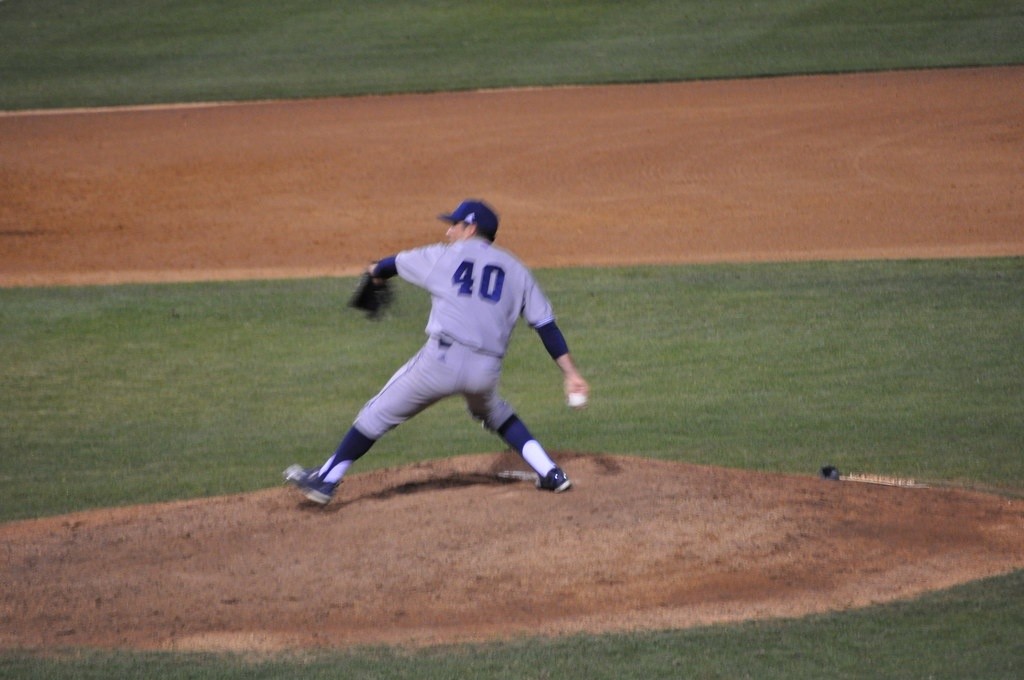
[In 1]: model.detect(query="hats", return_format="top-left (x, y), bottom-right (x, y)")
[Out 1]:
top-left (438, 201), bottom-right (497, 235)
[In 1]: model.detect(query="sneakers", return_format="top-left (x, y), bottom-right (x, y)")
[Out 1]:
top-left (535, 467), bottom-right (571, 493)
top-left (283, 464), bottom-right (334, 505)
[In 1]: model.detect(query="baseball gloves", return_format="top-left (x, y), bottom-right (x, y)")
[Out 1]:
top-left (345, 272), bottom-right (394, 314)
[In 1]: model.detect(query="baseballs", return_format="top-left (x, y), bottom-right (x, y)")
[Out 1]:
top-left (564, 391), bottom-right (589, 410)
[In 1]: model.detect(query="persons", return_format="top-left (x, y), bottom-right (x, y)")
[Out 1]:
top-left (284, 198), bottom-right (588, 502)
